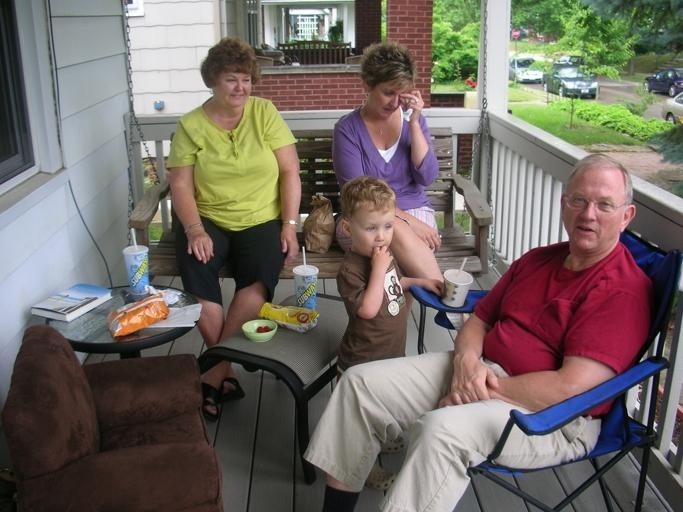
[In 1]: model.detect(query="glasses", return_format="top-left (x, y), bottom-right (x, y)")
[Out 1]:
top-left (563, 193), bottom-right (627, 215)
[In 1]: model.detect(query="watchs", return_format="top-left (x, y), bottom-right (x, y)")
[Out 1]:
top-left (281, 219), bottom-right (297, 227)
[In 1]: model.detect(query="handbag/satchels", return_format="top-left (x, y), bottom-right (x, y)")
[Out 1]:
top-left (302, 194), bottom-right (335, 254)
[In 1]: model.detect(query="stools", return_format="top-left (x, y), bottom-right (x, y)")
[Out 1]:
top-left (192, 292), bottom-right (356, 485)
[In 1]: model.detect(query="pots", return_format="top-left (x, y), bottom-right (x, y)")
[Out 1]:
top-left (240, 319), bottom-right (278, 343)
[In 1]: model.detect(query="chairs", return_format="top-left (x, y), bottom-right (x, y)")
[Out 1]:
top-left (410, 230), bottom-right (683, 510)
top-left (0, 323), bottom-right (226, 512)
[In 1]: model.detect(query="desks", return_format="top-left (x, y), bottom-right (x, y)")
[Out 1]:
top-left (44, 280), bottom-right (197, 359)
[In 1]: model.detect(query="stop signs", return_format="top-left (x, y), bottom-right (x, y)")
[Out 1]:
top-left (511, 27), bottom-right (520, 39)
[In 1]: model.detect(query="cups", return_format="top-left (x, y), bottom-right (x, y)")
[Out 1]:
top-left (291, 264), bottom-right (319, 310)
top-left (440, 269), bottom-right (473, 309)
top-left (291, 62), bottom-right (299, 67)
top-left (121, 244), bottom-right (150, 297)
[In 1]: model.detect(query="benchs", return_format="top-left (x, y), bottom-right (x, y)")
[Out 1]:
top-left (276, 40), bottom-right (353, 65)
top-left (126, 126), bottom-right (494, 279)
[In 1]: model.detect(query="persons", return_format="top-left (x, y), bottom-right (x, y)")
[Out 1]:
top-left (171, 39), bottom-right (302, 421)
top-left (335, 177), bottom-right (442, 489)
top-left (332, 43), bottom-right (442, 281)
top-left (318, 155), bottom-right (652, 512)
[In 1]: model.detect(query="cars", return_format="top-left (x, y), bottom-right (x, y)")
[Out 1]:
top-left (643, 68), bottom-right (682, 98)
top-left (553, 56), bottom-right (584, 65)
top-left (508, 57), bottom-right (545, 84)
top-left (661, 92), bottom-right (682, 126)
top-left (542, 64), bottom-right (599, 98)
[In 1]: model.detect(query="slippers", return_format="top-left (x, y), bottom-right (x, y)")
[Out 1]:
top-left (201, 377), bottom-right (245, 422)
